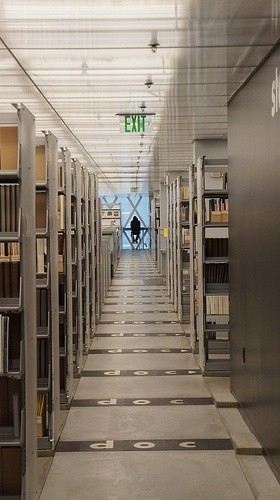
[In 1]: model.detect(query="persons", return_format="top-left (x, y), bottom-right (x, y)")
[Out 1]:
top-left (131, 216), bottom-right (140, 243)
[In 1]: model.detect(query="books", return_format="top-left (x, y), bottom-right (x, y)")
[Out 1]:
top-left (180, 171), bottom-right (231, 359)
top-left (1, 125), bottom-right (96, 496)
top-left (155, 207), bottom-right (160, 227)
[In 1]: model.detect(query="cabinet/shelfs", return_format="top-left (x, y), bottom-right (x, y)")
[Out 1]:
top-left (152, 154), bottom-right (229, 377)
top-left (0, 100), bottom-right (120, 500)
